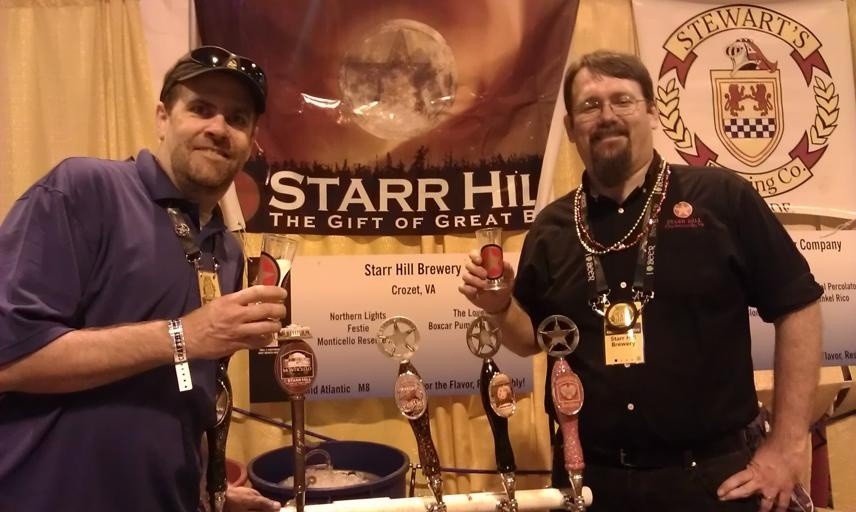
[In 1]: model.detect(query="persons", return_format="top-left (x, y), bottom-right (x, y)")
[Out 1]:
top-left (456, 48), bottom-right (826, 512)
top-left (0, 44), bottom-right (290, 512)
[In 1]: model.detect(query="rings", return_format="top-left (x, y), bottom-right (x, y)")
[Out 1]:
top-left (761, 492), bottom-right (778, 501)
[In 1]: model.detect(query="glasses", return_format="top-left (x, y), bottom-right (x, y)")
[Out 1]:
top-left (159, 44), bottom-right (268, 99)
top-left (571, 94), bottom-right (645, 121)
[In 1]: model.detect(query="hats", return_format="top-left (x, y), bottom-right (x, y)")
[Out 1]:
top-left (159, 47), bottom-right (267, 113)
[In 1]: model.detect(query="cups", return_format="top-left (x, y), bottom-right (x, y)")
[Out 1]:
top-left (473, 225), bottom-right (507, 292)
top-left (250, 232), bottom-right (299, 286)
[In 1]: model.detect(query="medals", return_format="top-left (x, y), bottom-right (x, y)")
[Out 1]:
top-left (606, 302), bottom-right (635, 328)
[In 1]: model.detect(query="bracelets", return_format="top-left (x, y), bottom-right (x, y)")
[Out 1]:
top-left (168, 317), bottom-right (193, 392)
top-left (488, 297), bottom-right (512, 315)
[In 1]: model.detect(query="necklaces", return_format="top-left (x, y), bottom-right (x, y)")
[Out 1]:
top-left (573, 160), bottom-right (673, 255)
